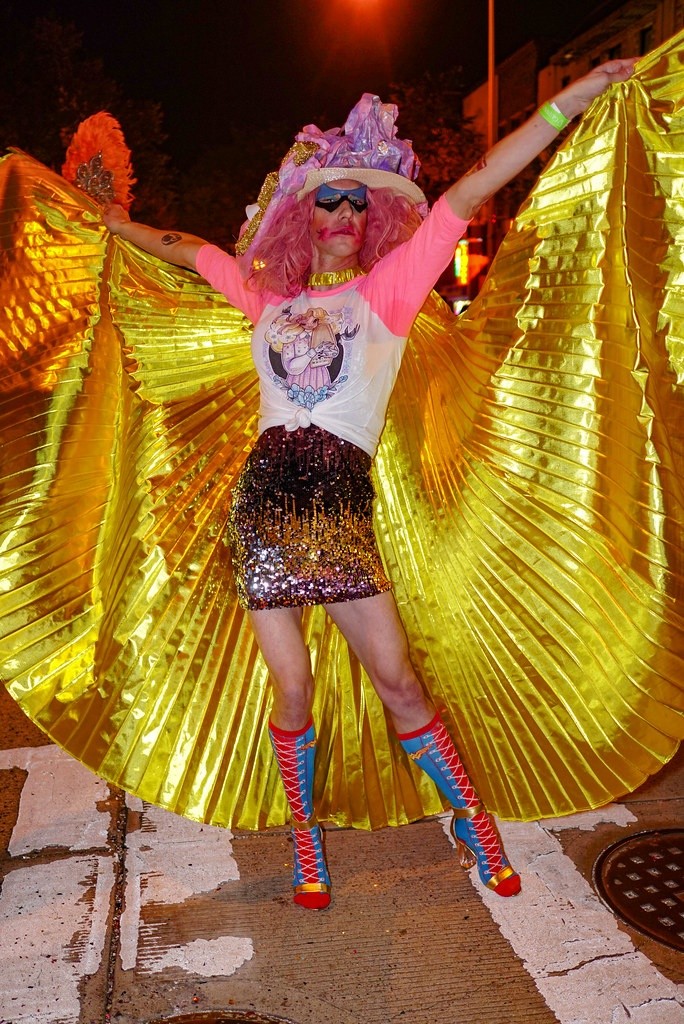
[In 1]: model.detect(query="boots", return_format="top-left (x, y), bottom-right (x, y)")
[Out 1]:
top-left (269, 713), bottom-right (332, 911)
top-left (396, 709), bottom-right (522, 897)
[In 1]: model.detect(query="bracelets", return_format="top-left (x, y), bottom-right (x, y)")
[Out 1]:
top-left (539, 99), bottom-right (572, 133)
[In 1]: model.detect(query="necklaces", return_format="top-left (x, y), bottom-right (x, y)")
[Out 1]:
top-left (307, 265), bottom-right (367, 289)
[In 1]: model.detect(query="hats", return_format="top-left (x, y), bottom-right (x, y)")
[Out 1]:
top-left (278, 92), bottom-right (429, 206)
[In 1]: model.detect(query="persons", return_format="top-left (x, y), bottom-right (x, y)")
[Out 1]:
top-left (99, 53), bottom-right (641, 908)
